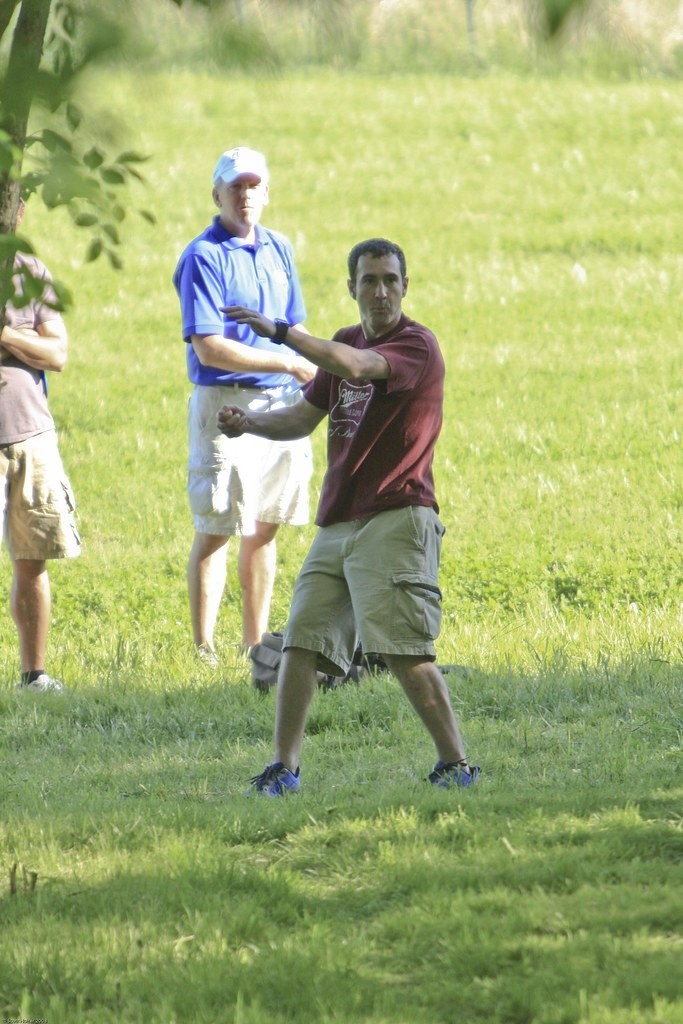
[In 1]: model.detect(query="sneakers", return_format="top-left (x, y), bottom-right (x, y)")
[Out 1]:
top-left (246, 762), bottom-right (300, 797)
top-left (425, 761), bottom-right (481, 789)
top-left (15, 675), bottom-right (63, 694)
top-left (194, 649), bottom-right (220, 670)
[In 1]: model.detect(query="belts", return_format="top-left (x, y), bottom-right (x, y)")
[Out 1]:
top-left (227, 383), bottom-right (271, 390)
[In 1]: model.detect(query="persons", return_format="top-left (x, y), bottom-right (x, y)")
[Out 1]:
top-left (216, 237), bottom-right (479, 799)
top-left (0, 197), bottom-right (82, 693)
top-left (174, 147), bottom-right (313, 667)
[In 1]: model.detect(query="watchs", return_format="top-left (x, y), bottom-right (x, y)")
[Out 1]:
top-left (271, 318), bottom-right (289, 344)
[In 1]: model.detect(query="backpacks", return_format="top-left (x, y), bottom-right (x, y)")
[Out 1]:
top-left (248, 632), bottom-right (390, 694)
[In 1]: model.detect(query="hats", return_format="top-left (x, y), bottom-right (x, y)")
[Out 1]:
top-left (212, 146), bottom-right (270, 188)
